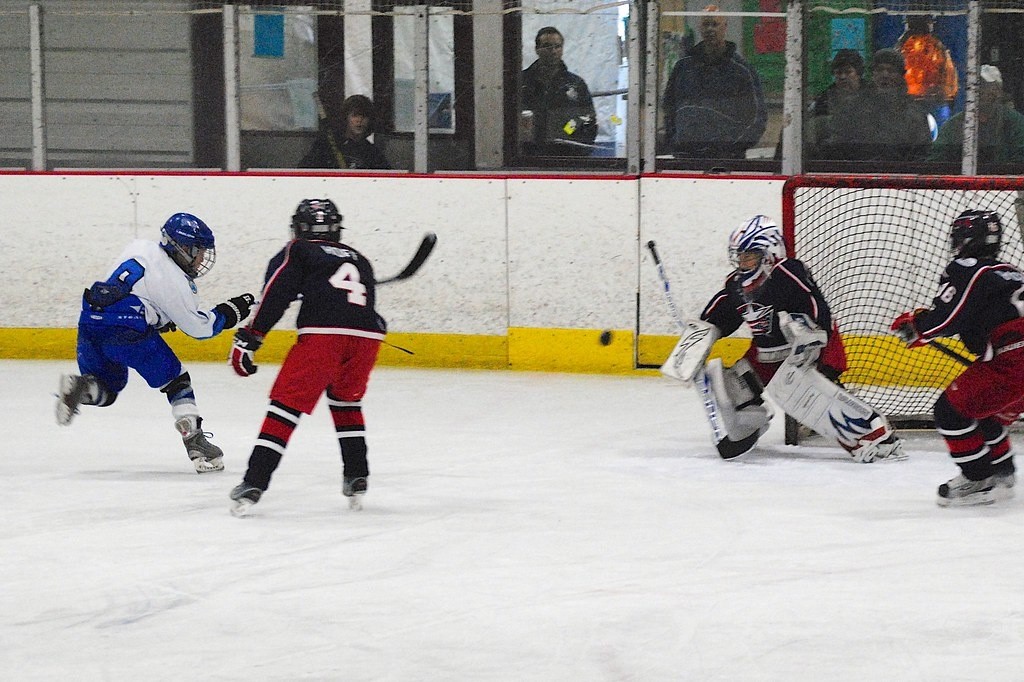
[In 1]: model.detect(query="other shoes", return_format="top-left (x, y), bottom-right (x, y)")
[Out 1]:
top-left (863, 435), bottom-right (910, 463)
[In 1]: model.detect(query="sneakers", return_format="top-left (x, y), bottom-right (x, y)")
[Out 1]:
top-left (342, 476), bottom-right (367, 511)
top-left (229, 481), bottom-right (262, 516)
top-left (55, 373), bottom-right (99, 426)
top-left (993, 474), bottom-right (1018, 501)
top-left (174, 416), bottom-right (224, 474)
top-left (937, 473), bottom-right (996, 505)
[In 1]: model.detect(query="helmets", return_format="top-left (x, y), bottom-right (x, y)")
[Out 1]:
top-left (291, 198), bottom-right (342, 242)
top-left (728, 214), bottom-right (786, 295)
top-left (951, 209), bottom-right (1003, 258)
top-left (159, 212), bottom-right (216, 279)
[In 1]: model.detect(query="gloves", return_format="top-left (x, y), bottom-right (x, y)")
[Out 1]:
top-left (890, 308), bottom-right (933, 350)
top-left (228, 332), bottom-right (263, 377)
top-left (159, 321), bottom-right (176, 333)
top-left (215, 293), bottom-right (256, 330)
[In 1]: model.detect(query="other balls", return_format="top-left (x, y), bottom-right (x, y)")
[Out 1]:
top-left (600, 330), bottom-right (612, 346)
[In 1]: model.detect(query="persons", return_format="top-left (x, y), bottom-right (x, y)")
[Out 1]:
top-left (227, 198), bottom-right (387, 503)
top-left (659, 215), bottom-right (898, 462)
top-left (297, 95), bottom-right (392, 170)
top-left (522, 26), bottom-right (599, 156)
top-left (661, 5), bottom-right (768, 159)
top-left (891, 209), bottom-right (1024, 499)
top-left (776, 49), bottom-right (1024, 163)
top-left (895, 7), bottom-right (959, 127)
top-left (57, 213), bottom-right (255, 461)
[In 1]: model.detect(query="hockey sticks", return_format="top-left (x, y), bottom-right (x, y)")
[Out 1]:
top-left (647, 240), bottom-right (761, 461)
top-left (906, 329), bottom-right (1024, 421)
top-left (383, 341), bottom-right (413, 354)
top-left (313, 91), bottom-right (347, 169)
top-left (251, 233), bottom-right (437, 306)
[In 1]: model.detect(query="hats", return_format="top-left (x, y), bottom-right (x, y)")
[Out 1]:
top-left (870, 48), bottom-right (905, 70)
top-left (832, 49), bottom-right (864, 76)
top-left (980, 64), bottom-right (1003, 86)
